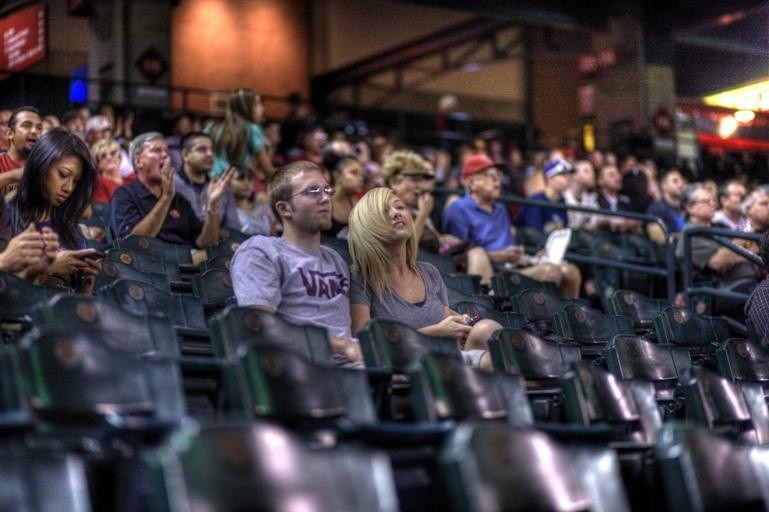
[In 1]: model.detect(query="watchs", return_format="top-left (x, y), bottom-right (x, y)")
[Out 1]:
top-left (203, 204), bottom-right (221, 215)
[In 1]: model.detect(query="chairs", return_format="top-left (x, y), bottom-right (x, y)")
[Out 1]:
top-left (1, 204), bottom-right (768, 512)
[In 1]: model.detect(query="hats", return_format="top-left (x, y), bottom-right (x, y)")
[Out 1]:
top-left (462, 156), bottom-right (506, 178)
top-left (543, 159), bottom-right (577, 177)
top-left (85, 115), bottom-right (112, 132)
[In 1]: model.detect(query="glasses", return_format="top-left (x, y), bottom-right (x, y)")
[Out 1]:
top-left (282, 185), bottom-right (336, 201)
top-left (689, 198), bottom-right (714, 205)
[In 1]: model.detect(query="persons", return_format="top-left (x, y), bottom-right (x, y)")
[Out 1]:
top-left (103, 103), bottom-right (135, 150)
top-left (108, 131), bottom-right (237, 251)
top-left (0, 127), bottom-right (103, 295)
top-left (347, 187), bottom-right (505, 375)
top-left (87, 134), bottom-right (138, 207)
top-left (717, 178), bottom-right (750, 233)
top-left (228, 161), bottom-right (276, 238)
top-left (648, 168), bottom-right (686, 244)
top-left (290, 124), bottom-right (328, 175)
top-left (330, 130), bottom-right (392, 164)
top-left (171, 131), bottom-right (244, 237)
top-left (384, 150), bottom-right (449, 254)
top-left (62, 111), bottom-right (83, 144)
top-left (680, 182), bottom-right (764, 291)
top-left (453, 114), bottom-right (768, 174)
top-left (441, 153), bottom-right (584, 303)
top-left (171, 115), bottom-right (194, 136)
top-left (228, 160), bottom-right (365, 368)
top-left (268, 91), bottom-right (315, 161)
top-left (562, 161), bottom-right (603, 230)
top-left (518, 159), bottom-right (580, 236)
top-left (595, 162), bottom-right (643, 229)
top-left (82, 114), bottom-right (112, 148)
top-left (0, 105), bottom-right (45, 202)
top-left (740, 186), bottom-right (769, 255)
top-left (201, 87), bottom-right (273, 179)
